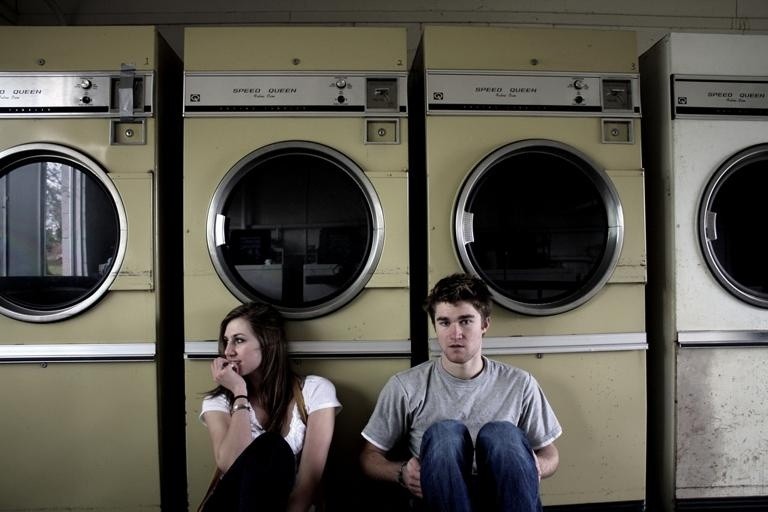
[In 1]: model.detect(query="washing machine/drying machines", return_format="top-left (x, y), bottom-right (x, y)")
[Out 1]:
top-left (185, 26), bottom-right (412, 509)
top-left (639, 33), bottom-right (768, 510)
top-left (1, 25), bottom-right (182, 512)
top-left (410, 25), bottom-right (649, 510)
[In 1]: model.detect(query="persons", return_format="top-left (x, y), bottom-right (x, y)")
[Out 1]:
top-left (357, 272), bottom-right (563, 511)
top-left (198, 301), bottom-right (344, 511)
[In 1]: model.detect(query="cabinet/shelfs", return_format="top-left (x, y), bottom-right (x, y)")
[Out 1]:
top-left (225, 189), bottom-right (368, 268)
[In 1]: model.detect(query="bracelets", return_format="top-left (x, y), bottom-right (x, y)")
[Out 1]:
top-left (230, 394), bottom-right (249, 408)
top-left (398, 461), bottom-right (408, 490)
top-left (229, 402), bottom-right (251, 416)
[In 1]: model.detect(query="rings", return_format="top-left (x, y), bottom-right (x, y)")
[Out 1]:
top-left (228, 362), bottom-right (233, 367)
